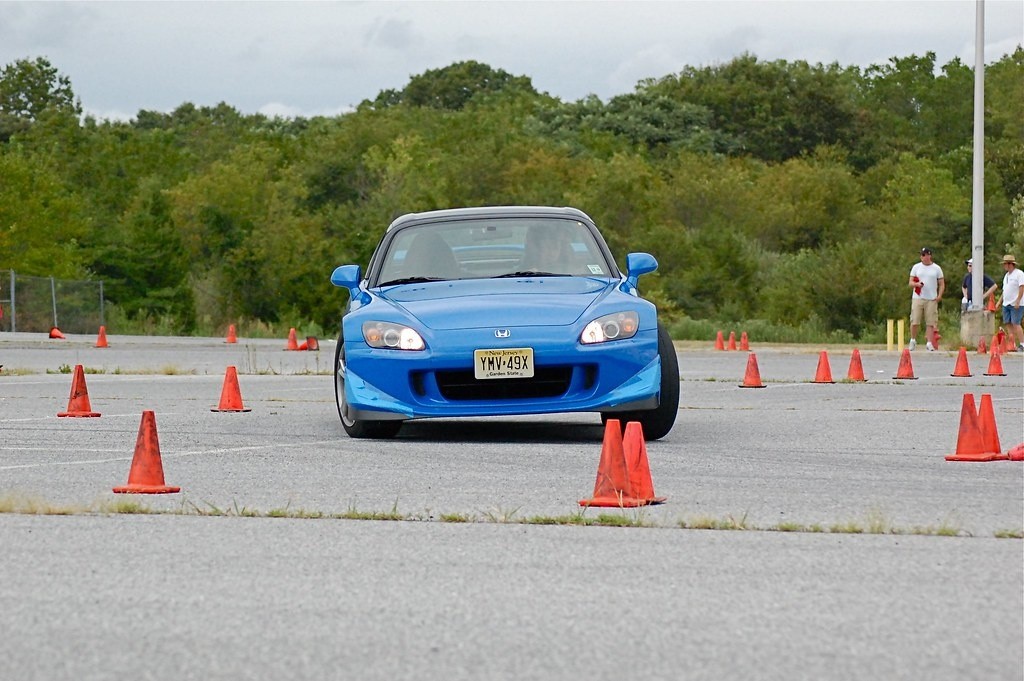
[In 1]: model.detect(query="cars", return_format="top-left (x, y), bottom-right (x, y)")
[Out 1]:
top-left (329, 206), bottom-right (680, 440)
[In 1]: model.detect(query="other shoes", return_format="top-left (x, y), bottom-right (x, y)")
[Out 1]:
top-left (909, 340), bottom-right (917, 351)
top-left (1016, 345), bottom-right (1024, 351)
top-left (926, 343), bottom-right (935, 351)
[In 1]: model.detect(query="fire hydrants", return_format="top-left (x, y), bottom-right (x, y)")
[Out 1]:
top-left (931, 329), bottom-right (941, 350)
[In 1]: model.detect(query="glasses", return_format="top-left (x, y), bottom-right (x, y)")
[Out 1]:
top-left (920, 254), bottom-right (926, 256)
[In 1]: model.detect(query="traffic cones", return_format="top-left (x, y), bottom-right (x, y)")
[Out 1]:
top-left (977, 333), bottom-right (1018, 354)
top-left (48, 327), bottom-right (65, 339)
top-left (950, 346), bottom-right (973, 377)
top-left (738, 352), bottom-right (768, 388)
top-left (283, 327), bottom-right (319, 351)
top-left (712, 331), bottom-right (752, 351)
top-left (113, 411), bottom-right (182, 494)
top-left (582, 420), bottom-right (666, 507)
top-left (944, 393), bottom-right (1024, 462)
top-left (210, 366), bottom-right (252, 412)
top-left (893, 348), bottom-right (919, 380)
top-left (222, 325), bottom-right (237, 343)
top-left (94, 326), bottom-right (111, 348)
top-left (848, 349), bottom-right (869, 382)
top-left (56, 365), bottom-right (102, 417)
top-left (983, 347), bottom-right (1008, 376)
top-left (810, 352), bottom-right (836, 383)
top-left (983, 292), bottom-right (996, 312)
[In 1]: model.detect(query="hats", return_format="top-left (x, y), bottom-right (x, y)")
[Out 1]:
top-left (917, 248), bottom-right (932, 255)
top-left (965, 258), bottom-right (972, 264)
top-left (999, 255), bottom-right (1020, 265)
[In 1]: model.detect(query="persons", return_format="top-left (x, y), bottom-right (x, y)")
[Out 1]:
top-left (908, 247), bottom-right (945, 351)
top-left (996, 254), bottom-right (1024, 353)
top-left (521, 226), bottom-right (577, 274)
top-left (961, 258), bottom-right (998, 311)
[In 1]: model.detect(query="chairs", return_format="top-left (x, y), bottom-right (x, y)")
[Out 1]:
top-left (521, 228), bottom-right (575, 270)
top-left (403, 231), bottom-right (459, 276)
